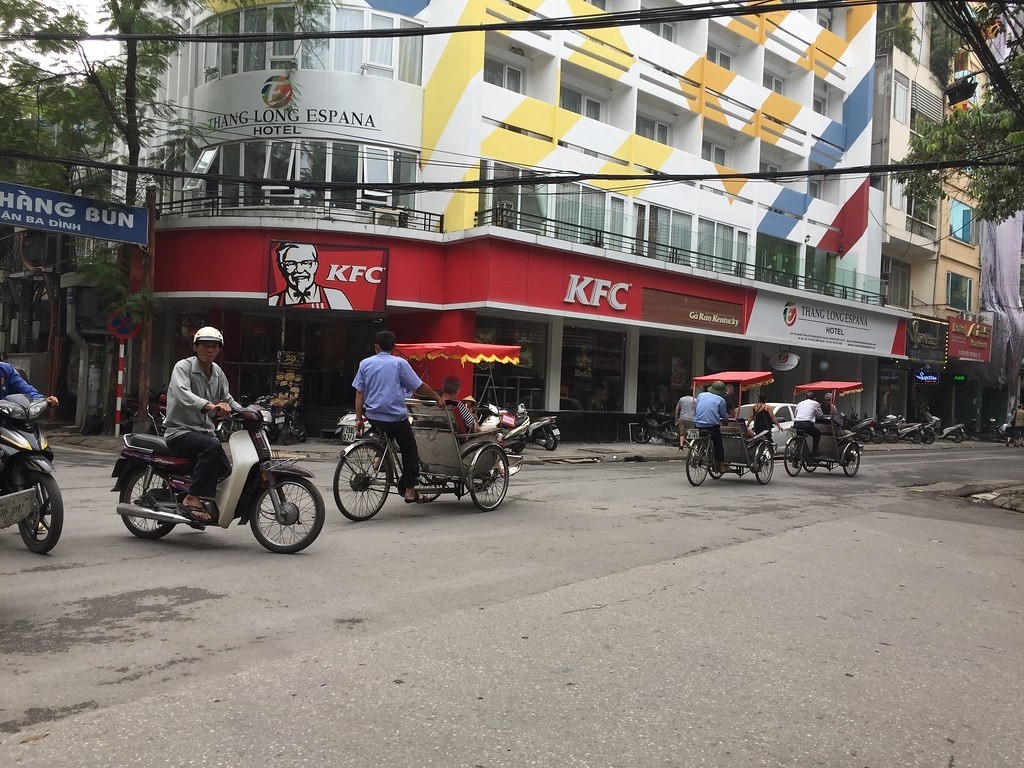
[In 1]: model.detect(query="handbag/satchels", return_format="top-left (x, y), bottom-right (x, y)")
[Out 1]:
top-left (1010, 416), bottom-right (1016, 428)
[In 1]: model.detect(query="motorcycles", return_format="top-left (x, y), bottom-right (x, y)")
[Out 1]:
top-left (921, 406), bottom-right (968, 445)
top-left (478, 402), bottom-right (531, 454)
top-left (512, 399), bottom-right (561, 451)
top-left (633, 403), bottom-right (684, 447)
top-left (838, 406), bottom-right (926, 444)
top-left (110, 403), bottom-right (326, 555)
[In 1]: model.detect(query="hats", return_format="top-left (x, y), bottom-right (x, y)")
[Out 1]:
top-left (462, 395), bottom-right (476, 403)
top-left (708, 381), bottom-right (728, 395)
top-left (824, 393), bottom-right (832, 400)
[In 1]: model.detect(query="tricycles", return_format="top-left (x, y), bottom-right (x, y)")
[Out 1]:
top-left (333, 342), bottom-right (525, 522)
top-left (685, 370), bottom-right (778, 487)
top-left (783, 381), bottom-right (864, 477)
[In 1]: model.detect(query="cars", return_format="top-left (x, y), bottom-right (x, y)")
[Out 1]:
top-left (717, 403), bottom-right (816, 462)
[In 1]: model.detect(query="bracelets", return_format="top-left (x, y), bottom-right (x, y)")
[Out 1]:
top-left (355, 418), bottom-right (362, 422)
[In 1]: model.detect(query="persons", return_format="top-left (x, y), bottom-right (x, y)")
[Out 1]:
top-left (0, 361), bottom-right (59, 407)
top-left (820, 393), bottom-right (853, 440)
top-left (793, 391), bottom-right (823, 450)
top-left (462, 395), bottom-right (476, 408)
top-left (693, 381), bottom-right (729, 472)
top-left (748, 394), bottom-right (783, 444)
top-left (163, 326), bottom-right (244, 522)
top-left (1006, 404), bottom-right (1024, 447)
top-left (352, 331), bottom-right (446, 503)
top-left (674, 388), bottom-right (696, 452)
top-left (724, 384), bottom-right (734, 404)
top-left (442, 377), bottom-right (517, 474)
top-left (726, 402), bottom-right (753, 438)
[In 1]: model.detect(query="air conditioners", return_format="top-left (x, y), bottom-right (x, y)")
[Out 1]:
top-left (373, 208), bottom-right (400, 226)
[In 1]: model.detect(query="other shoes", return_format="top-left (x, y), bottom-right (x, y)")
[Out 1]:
top-left (678, 446), bottom-right (683, 453)
top-left (811, 451), bottom-right (824, 456)
top-left (792, 462), bottom-right (799, 469)
top-left (1006, 442), bottom-right (1009, 447)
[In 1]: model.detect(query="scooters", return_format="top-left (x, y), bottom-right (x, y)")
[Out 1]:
top-left (146, 383), bottom-right (309, 446)
top-left (965, 414), bottom-right (1007, 443)
top-left (0, 393), bottom-right (64, 555)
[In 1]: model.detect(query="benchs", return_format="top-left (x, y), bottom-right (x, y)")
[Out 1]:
top-left (404, 399), bottom-right (500, 459)
top-left (804, 418), bottom-right (856, 446)
top-left (718, 417), bottom-right (770, 443)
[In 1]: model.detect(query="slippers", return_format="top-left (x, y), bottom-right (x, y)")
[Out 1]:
top-left (720, 465), bottom-right (732, 474)
top-left (178, 500), bottom-right (212, 524)
top-left (374, 464), bottom-right (384, 471)
top-left (405, 489), bottom-right (427, 503)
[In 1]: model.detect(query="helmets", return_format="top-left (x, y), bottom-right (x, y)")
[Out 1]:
top-left (193, 326), bottom-right (223, 346)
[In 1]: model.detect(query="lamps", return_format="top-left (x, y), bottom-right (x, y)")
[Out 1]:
top-left (840, 246), bottom-right (843, 251)
top-left (360, 63), bottom-right (368, 75)
top-left (205, 66), bottom-right (220, 80)
top-left (290, 58), bottom-right (298, 70)
top-left (804, 234), bottom-right (810, 243)
